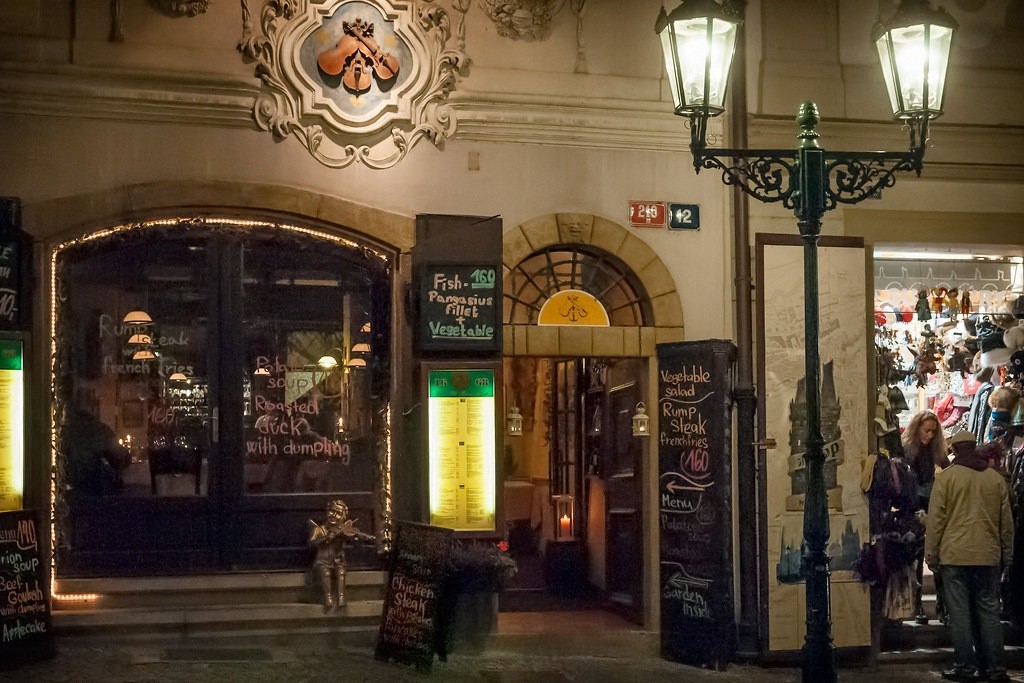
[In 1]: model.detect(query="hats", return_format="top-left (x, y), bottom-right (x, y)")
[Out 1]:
top-left (952, 431), bottom-right (977, 443)
top-left (943, 294), bottom-right (1024, 428)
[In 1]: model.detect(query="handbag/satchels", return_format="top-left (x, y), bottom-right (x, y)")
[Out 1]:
top-left (869, 453), bottom-right (919, 509)
top-left (885, 572), bottom-right (916, 621)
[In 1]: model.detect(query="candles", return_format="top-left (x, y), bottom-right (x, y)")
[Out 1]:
top-left (561, 515), bottom-right (570, 538)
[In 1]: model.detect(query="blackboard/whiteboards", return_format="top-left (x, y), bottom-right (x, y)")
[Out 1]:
top-left (416, 259), bottom-right (503, 354)
top-left (0, 512), bottom-right (57, 672)
top-left (653, 341), bottom-right (735, 672)
top-left (374, 520), bottom-right (456, 672)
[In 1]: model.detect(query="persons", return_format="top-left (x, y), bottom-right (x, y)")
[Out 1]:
top-left (904, 410), bottom-right (1014, 682)
top-left (308, 500), bottom-right (361, 610)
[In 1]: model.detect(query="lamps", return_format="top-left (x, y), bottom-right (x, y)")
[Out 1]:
top-left (318, 347), bottom-right (343, 369)
top-left (345, 321), bottom-right (371, 369)
top-left (128, 334), bottom-right (153, 344)
top-left (122, 307), bottom-right (152, 324)
top-left (170, 366), bottom-right (187, 381)
top-left (633, 402), bottom-right (651, 436)
top-left (508, 401), bottom-right (522, 436)
top-left (132, 350), bottom-right (155, 360)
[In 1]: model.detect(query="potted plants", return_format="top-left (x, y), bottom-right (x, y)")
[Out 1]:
top-left (434, 539), bottom-right (519, 656)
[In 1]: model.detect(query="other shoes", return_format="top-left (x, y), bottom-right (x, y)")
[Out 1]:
top-left (943, 667), bottom-right (983, 680)
top-left (984, 671), bottom-right (1012, 682)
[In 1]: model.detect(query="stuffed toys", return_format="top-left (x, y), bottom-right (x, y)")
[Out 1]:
top-left (914, 286), bottom-right (971, 322)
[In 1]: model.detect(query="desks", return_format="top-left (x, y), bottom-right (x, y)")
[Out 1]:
top-left (504, 481), bottom-right (537, 543)
